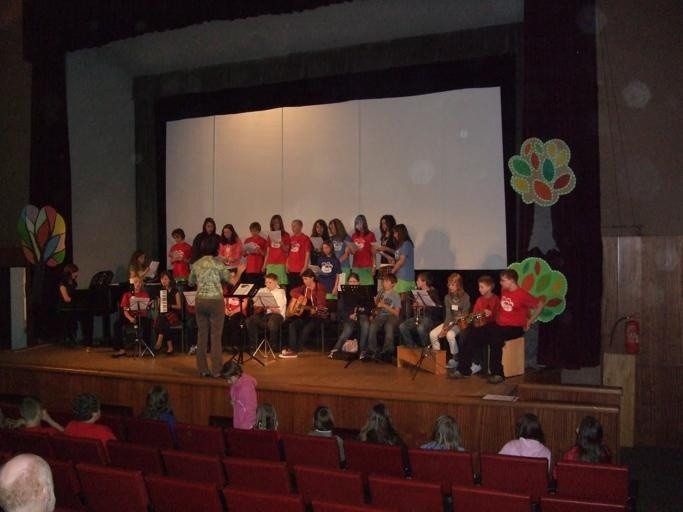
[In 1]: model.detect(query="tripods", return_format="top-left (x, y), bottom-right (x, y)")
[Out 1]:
top-left (407, 290), bottom-right (455, 381)
top-left (249, 294), bottom-right (280, 360)
top-left (124, 298), bottom-right (156, 358)
top-left (225, 280), bottom-right (265, 367)
top-left (340, 285), bottom-right (386, 368)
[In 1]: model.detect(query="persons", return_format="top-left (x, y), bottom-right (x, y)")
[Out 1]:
top-left (221, 361), bottom-right (257, 430)
top-left (64, 392), bottom-right (117, 441)
top-left (112, 214), bottom-right (414, 363)
top-left (399, 268), bottom-right (544, 379)
top-left (138, 385), bottom-right (176, 439)
top-left (554, 416), bottom-right (613, 479)
top-left (0, 453), bottom-right (56, 512)
top-left (255, 402), bottom-right (278, 432)
top-left (498, 414), bottom-right (551, 471)
top-left (308, 407), bottom-right (345, 463)
top-left (13, 397), bottom-right (64, 436)
top-left (419, 415), bottom-right (466, 451)
top-left (187, 237), bottom-right (247, 379)
top-left (58, 263), bottom-right (92, 346)
top-left (356, 403), bottom-right (410, 477)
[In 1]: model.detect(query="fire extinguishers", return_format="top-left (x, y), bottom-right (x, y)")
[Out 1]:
top-left (608, 312), bottom-right (640, 354)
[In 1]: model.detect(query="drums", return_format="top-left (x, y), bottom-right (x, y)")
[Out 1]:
top-left (453, 314), bottom-right (469, 330)
top-left (471, 311), bottom-right (485, 327)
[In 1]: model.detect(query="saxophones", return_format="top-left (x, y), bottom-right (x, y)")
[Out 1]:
top-left (370, 286), bottom-right (386, 316)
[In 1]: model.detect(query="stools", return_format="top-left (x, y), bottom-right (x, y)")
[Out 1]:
top-left (396, 343), bottom-right (447, 376)
top-left (501, 336), bottom-right (525, 378)
top-left (61, 296), bottom-right (404, 359)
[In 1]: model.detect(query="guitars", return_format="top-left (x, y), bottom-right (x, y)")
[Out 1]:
top-left (285, 296), bottom-right (328, 317)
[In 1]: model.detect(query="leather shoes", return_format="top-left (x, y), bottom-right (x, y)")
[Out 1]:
top-left (200, 370), bottom-right (211, 377)
top-left (112, 352), bottom-right (126, 358)
top-left (166, 351), bottom-right (173, 355)
top-left (152, 347), bottom-right (160, 354)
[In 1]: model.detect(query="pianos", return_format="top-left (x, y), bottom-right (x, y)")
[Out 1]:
top-left (57, 271), bottom-right (129, 345)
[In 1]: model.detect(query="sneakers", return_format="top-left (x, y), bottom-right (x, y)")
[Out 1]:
top-left (444, 359), bottom-right (481, 378)
top-left (489, 375), bottom-right (503, 384)
top-left (279, 351), bottom-right (297, 358)
